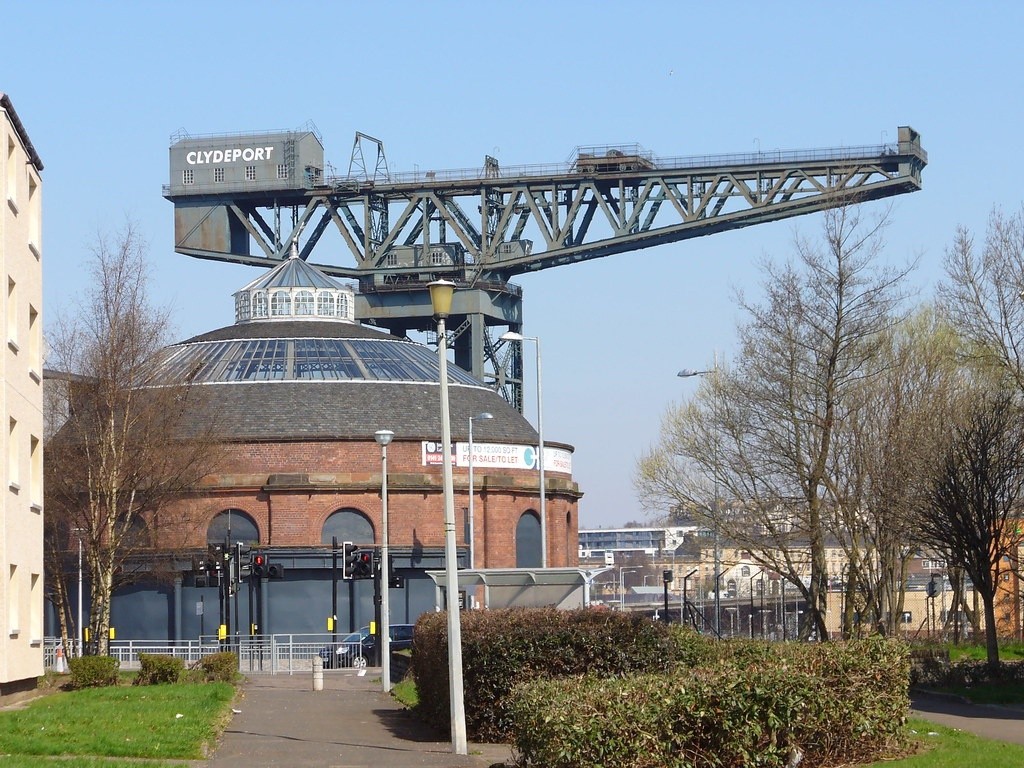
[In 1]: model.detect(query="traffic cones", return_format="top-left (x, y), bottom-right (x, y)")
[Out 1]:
top-left (56, 647), bottom-right (65, 677)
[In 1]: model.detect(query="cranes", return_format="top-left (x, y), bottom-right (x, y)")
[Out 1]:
top-left (160, 123), bottom-right (929, 410)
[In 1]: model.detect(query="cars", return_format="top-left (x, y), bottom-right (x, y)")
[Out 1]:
top-left (319, 623), bottom-right (419, 670)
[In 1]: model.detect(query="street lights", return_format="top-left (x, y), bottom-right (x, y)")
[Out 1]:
top-left (675, 369), bottom-right (721, 636)
top-left (421, 276), bottom-right (467, 755)
top-left (71, 527), bottom-right (88, 659)
top-left (498, 332), bottom-right (548, 569)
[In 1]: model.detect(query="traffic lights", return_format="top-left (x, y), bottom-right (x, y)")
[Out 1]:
top-left (208, 560), bottom-right (218, 589)
top-left (342, 541), bottom-right (359, 581)
top-left (359, 550), bottom-right (373, 576)
top-left (251, 553), bottom-right (284, 580)
top-left (195, 558), bottom-right (208, 588)
